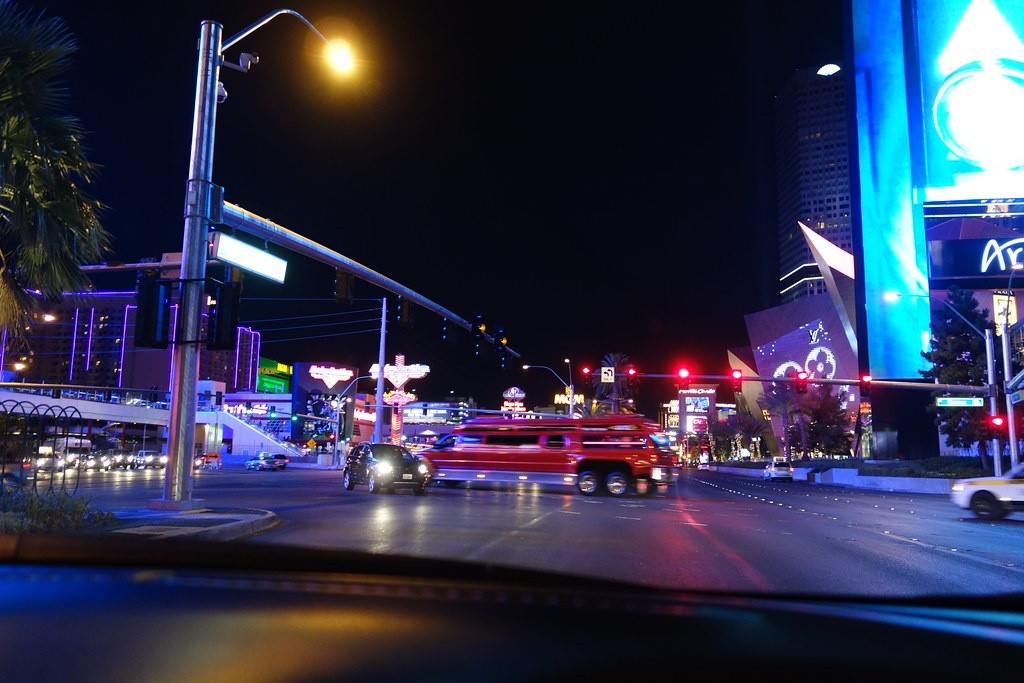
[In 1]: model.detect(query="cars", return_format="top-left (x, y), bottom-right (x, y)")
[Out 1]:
top-left (17, 450), bottom-right (223, 473)
top-left (950, 460), bottom-right (1024, 522)
top-left (763, 461), bottom-right (794, 482)
top-left (696, 463), bottom-right (711, 473)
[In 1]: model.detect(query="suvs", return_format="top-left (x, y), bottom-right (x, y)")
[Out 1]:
top-left (343, 442), bottom-right (431, 496)
top-left (243, 452), bottom-right (290, 472)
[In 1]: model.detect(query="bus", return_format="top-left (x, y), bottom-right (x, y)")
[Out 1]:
top-left (411, 411), bottom-right (688, 499)
top-left (38, 432), bottom-right (93, 461)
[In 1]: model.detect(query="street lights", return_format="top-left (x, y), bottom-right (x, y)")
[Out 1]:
top-left (882, 285), bottom-right (1003, 480)
top-left (145, 5), bottom-right (363, 514)
top-left (523, 358), bottom-right (574, 418)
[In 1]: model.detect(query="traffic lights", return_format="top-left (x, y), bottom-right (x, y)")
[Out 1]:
top-left (472, 311), bottom-right (487, 360)
top-left (246, 401), bottom-right (252, 416)
top-left (270, 406), bottom-right (277, 419)
top-left (329, 432), bottom-right (335, 443)
top-left (795, 371), bottom-right (808, 394)
top-left (495, 328), bottom-right (508, 371)
top-left (730, 368), bottom-right (742, 393)
top-left (291, 410), bottom-right (297, 420)
top-left (678, 364), bottom-right (689, 382)
top-left (582, 365), bottom-right (591, 382)
top-left (860, 373), bottom-right (872, 394)
top-left (396, 294), bottom-right (412, 328)
top-left (627, 363), bottom-right (638, 389)
top-left (442, 316), bottom-right (455, 346)
top-left (991, 414), bottom-right (1003, 430)
